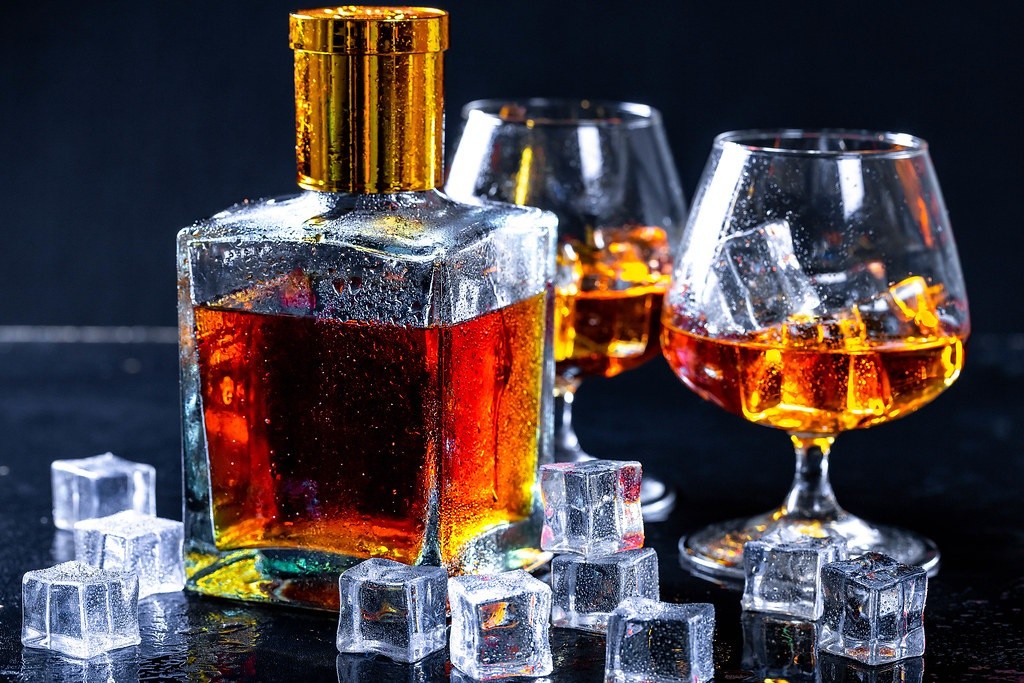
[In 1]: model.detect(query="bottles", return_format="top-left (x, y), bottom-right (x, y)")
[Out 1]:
top-left (177, 4), bottom-right (560, 611)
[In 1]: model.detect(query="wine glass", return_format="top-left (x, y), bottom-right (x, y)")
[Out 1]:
top-left (661, 130), bottom-right (971, 595)
top-left (441, 94), bottom-right (688, 521)
